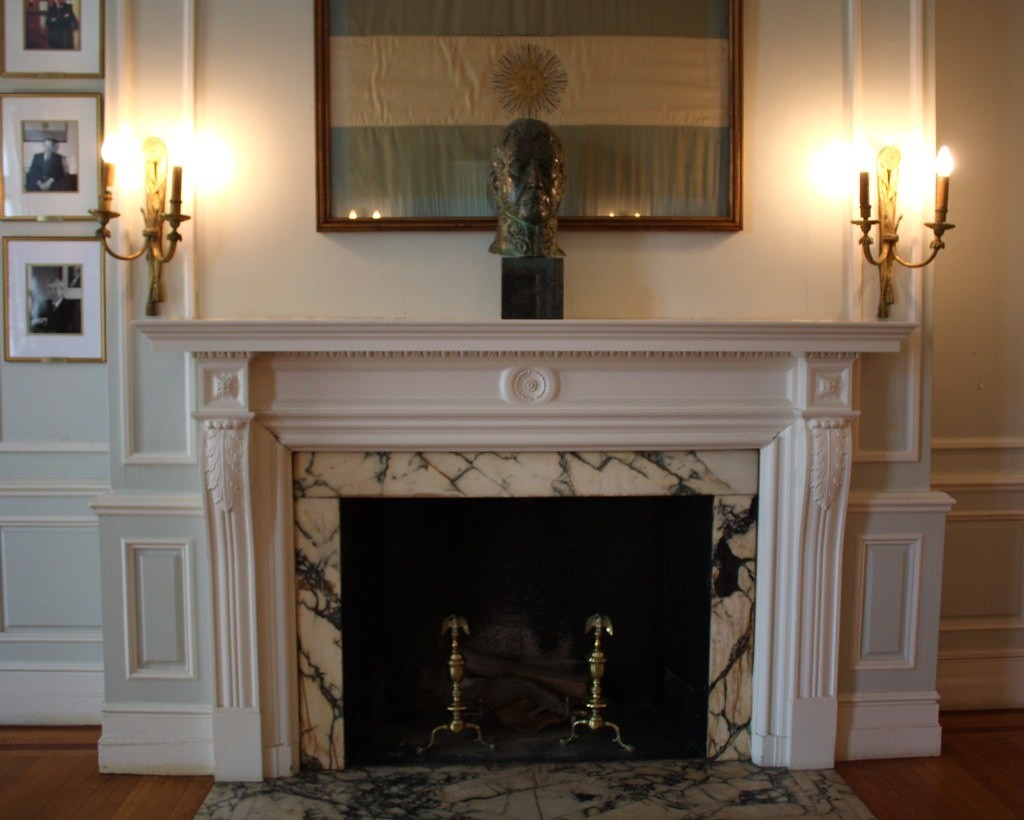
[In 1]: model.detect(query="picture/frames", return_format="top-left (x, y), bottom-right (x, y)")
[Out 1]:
top-left (312, 3), bottom-right (744, 234)
top-left (0, 0), bottom-right (107, 365)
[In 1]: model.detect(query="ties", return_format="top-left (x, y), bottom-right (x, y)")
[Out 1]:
top-left (44, 155), bottom-right (48, 179)
top-left (51, 303), bottom-right (56, 314)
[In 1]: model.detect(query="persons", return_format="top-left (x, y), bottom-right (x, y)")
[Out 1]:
top-left (26, 139), bottom-right (69, 191)
top-left (487, 118), bottom-right (567, 258)
top-left (35, 279), bottom-right (79, 332)
top-left (46, 0), bottom-right (79, 49)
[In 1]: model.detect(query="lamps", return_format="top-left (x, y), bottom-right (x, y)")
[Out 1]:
top-left (89, 113), bottom-right (232, 316)
top-left (854, 140), bottom-right (958, 321)
